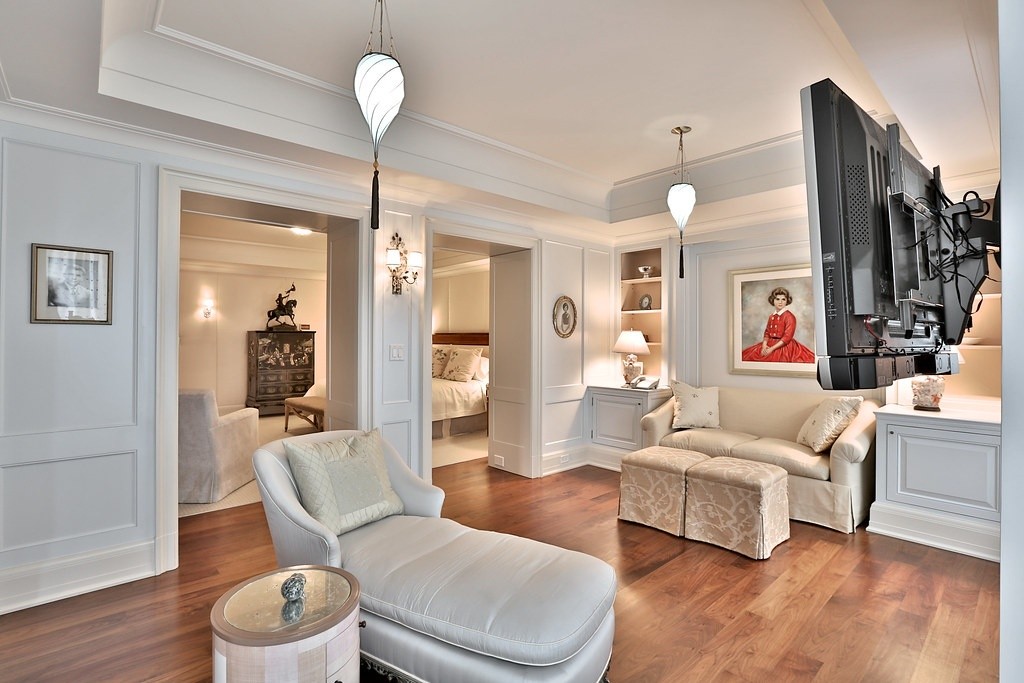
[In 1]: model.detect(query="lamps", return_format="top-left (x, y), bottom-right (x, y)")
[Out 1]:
top-left (612, 327), bottom-right (650, 385)
top-left (386, 232), bottom-right (424, 296)
top-left (667, 126), bottom-right (696, 279)
top-left (353, 0), bottom-right (405, 229)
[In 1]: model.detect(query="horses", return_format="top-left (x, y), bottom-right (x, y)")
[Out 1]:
top-left (267, 299), bottom-right (297, 328)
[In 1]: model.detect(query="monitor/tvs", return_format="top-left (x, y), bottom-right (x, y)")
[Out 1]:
top-left (800, 77), bottom-right (1001, 390)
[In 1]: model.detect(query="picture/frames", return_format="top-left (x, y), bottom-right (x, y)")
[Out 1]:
top-left (30, 243), bottom-right (113, 326)
top-left (552, 296), bottom-right (578, 338)
top-left (726, 263), bottom-right (817, 379)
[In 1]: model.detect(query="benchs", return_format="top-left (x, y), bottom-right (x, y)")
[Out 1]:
top-left (284, 396), bottom-right (326, 433)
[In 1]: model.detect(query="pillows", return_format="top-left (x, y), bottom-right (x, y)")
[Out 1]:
top-left (670, 379), bottom-right (721, 429)
top-left (430, 344), bottom-right (490, 383)
top-left (280, 428), bottom-right (404, 537)
top-left (796, 396), bottom-right (863, 453)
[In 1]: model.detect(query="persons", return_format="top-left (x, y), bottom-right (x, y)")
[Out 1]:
top-left (275, 293), bottom-right (289, 314)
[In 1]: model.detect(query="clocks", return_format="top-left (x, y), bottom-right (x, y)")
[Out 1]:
top-left (638, 293), bottom-right (652, 310)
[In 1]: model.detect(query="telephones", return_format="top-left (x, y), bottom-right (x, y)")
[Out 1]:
top-left (630, 375), bottom-right (660, 389)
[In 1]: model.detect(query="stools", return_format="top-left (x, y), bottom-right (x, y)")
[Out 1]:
top-left (617, 446), bottom-right (790, 560)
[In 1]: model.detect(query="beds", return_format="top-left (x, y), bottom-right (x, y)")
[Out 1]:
top-left (304, 332), bottom-right (490, 442)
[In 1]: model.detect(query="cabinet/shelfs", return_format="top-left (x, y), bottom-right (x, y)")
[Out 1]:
top-left (865, 246), bottom-right (1001, 565)
top-left (618, 245), bottom-right (662, 346)
top-left (246, 330), bottom-right (317, 415)
top-left (586, 385), bottom-right (672, 473)
top-left (209, 564), bottom-right (366, 683)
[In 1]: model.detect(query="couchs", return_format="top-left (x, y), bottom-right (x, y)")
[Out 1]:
top-left (178, 388), bottom-right (259, 503)
top-left (640, 387), bottom-right (882, 534)
top-left (252, 428), bottom-right (616, 683)
